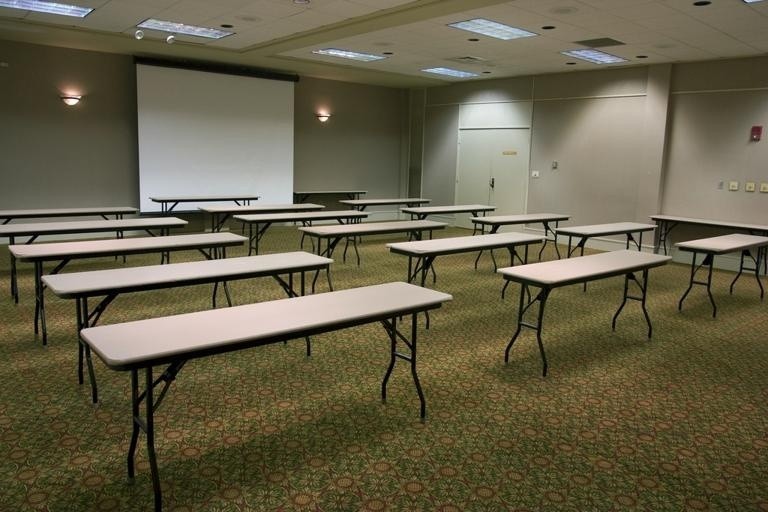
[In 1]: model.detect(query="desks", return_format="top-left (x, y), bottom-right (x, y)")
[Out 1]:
top-left (676, 232), bottom-right (768, 318)
top-left (401, 204), bottom-right (495, 242)
top-left (649, 214), bottom-right (768, 276)
top-left (298, 220), bottom-right (448, 294)
top-left (1, 207), bottom-right (136, 262)
top-left (553, 221), bottom-right (657, 290)
top-left (471, 213), bottom-right (571, 273)
top-left (80, 282), bottom-right (451, 511)
top-left (337, 197), bottom-right (432, 244)
top-left (148, 195), bottom-right (260, 237)
top-left (1, 217), bottom-right (188, 303)
top-left (497, 249), bottom-right (672, 377)
top-left (197, 203), bottom-right (325, 253)
top-left (233, 210), bottom-right (369, 266)
top-left (386, 232), bottom-right (547, 330)
top-left (8, 231), bottom-right (248, 344)
top-left (294, 190), bottom-right (367, 223)
top-left (41, 251), bottom-right (334, 402)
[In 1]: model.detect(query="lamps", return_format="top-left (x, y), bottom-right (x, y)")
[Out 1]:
top-left (58, 94), bottom-right (82, 107)
top-left (135, 29), bottom-right (144, 40)
top-left (166, 35), bottom-right (175, 45)
top-left (316, 112), bottom-right (331, 122)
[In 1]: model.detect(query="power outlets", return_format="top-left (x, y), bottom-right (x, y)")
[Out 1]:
top-left (729, 181), bottom-right (738, 190)
top-left (532, 170), bottom-right (539, 177)
top-left (746, 182), bottom-right (755, 192)
top-left (760, 183), bottom-right (768, 192)
